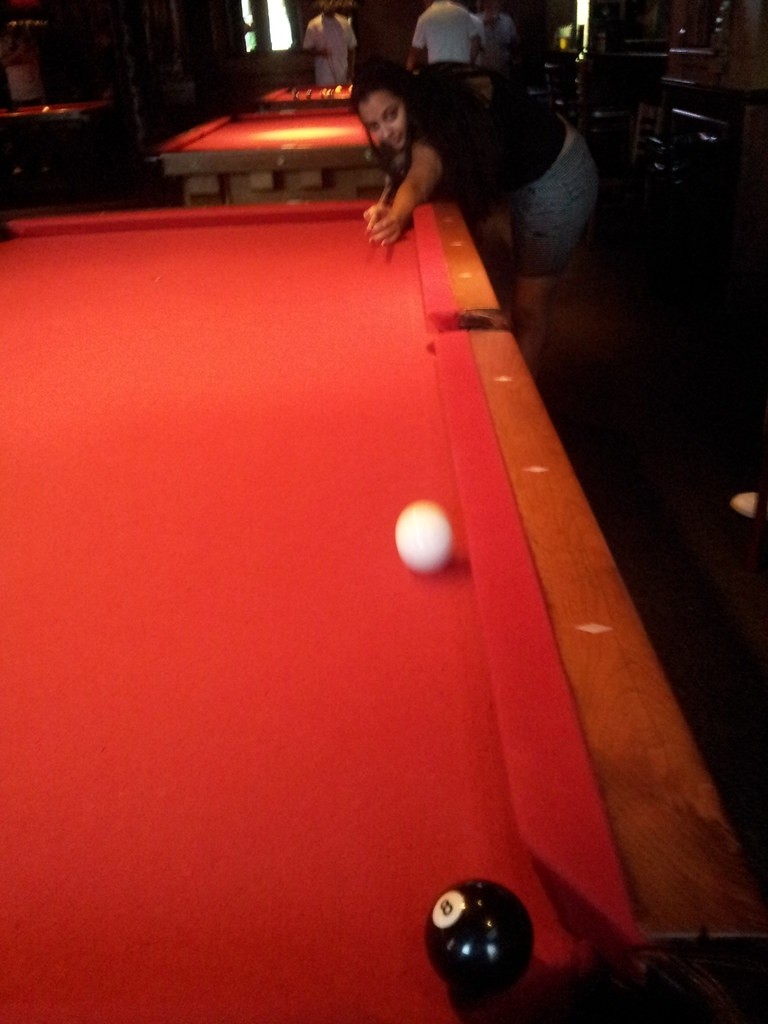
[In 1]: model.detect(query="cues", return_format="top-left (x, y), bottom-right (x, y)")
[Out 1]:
top-left (326, 56), bottom-right (337, 84)
top-left (366, 180), bottom-right (395, 232)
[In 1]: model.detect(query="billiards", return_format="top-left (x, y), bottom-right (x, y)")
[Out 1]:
top-left (424, 875), bottom-right (536, 997)
top-left (295, 84), bottom-right (354, 99)
top-left (396, 498), bottom-right (453, 575)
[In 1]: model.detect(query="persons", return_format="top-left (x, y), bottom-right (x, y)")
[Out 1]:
top-left (352, 56), bottom-right (599, 383)
top-left (406, 0), bottom-right (520, 76)
top-left (302, 0), bottom-right (358, 85)
top-left (0, 26), bottom-right (46, 106)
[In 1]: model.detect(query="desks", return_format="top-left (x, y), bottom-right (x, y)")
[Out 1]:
top-left (0, 182), bottom-right (768, 1024)
top-left (146, 104), bottom-right (392, 205)
top-left (1, 102), bottom-right (108, 184)
top-left (260, 85), bottom-right (355, 109)
